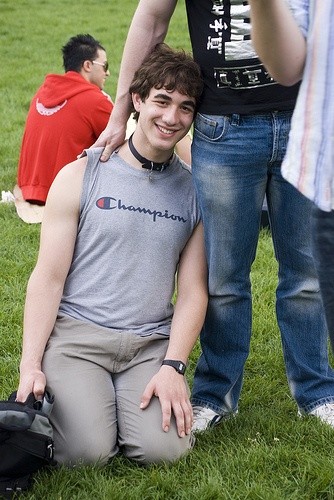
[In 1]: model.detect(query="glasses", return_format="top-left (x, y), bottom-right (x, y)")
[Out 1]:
top-left (93, 61), bottom-right (109, 72)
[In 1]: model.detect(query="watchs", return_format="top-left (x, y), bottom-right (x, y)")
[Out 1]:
top-left (160, 360), bottom-right (186, 375)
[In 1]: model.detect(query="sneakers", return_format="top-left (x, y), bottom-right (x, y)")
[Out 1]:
top-left (191, 400), bottom-right (238, 434)
top-left (298, 400), bottom-right (333, 430)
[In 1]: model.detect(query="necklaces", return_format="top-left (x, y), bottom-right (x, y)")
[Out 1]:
top-left (127, 131), bottom-right (173, 171)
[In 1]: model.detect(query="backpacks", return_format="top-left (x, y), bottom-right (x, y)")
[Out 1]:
top-left (0, 388), bottom-right (57, 500)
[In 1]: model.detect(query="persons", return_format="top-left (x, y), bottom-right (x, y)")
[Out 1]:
top-left (17, 41), bottom-right (209, 468)
top-left (89, 1), bottom-right (334, 436)
top-left (246, 0), bottom-right (333, 357)
top-left (12, 34), bottom-right (116, 223)
